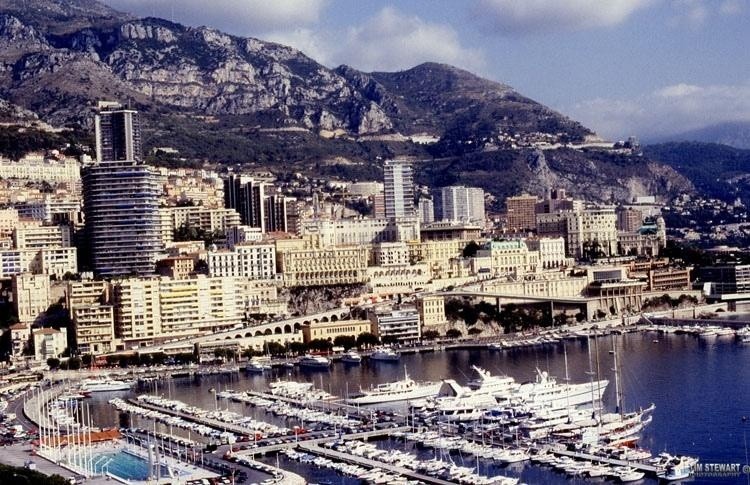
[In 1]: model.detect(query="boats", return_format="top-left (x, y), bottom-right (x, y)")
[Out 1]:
top-left (280, 427), bottom-right (645, 485)
top-left (643, 321), bottom-right (750, 347)
top-left (493, 337), bottom-right (561, 350)
top-left (271, 377), bottom-right (314, 399)
top-left (44, 389), bottom-right (361, 441)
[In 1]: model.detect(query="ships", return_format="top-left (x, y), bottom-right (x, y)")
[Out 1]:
top-left (77, 377), bottom-right (133, 393)
top-left (346, 354), bottom-right (610, 416)
top-left (557, 330), bottom-right (660, 441)
top-left (241, 345), bottom-right (402, 377)
